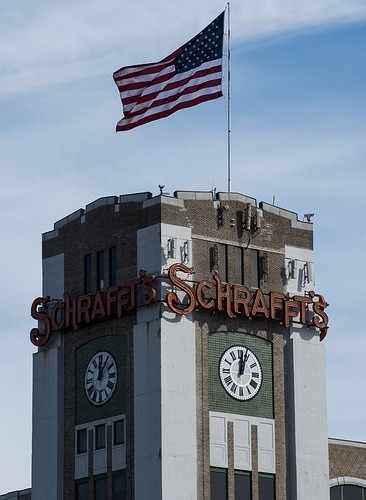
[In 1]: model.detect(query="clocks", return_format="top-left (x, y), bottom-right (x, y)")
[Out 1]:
top-left (84, 351), bottom-right (118, 406)
top-left (218, 345), bottom-right (264, 401)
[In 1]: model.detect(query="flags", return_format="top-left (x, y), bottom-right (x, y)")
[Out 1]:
top-left (114, 8), bottom-right (229, 133)
